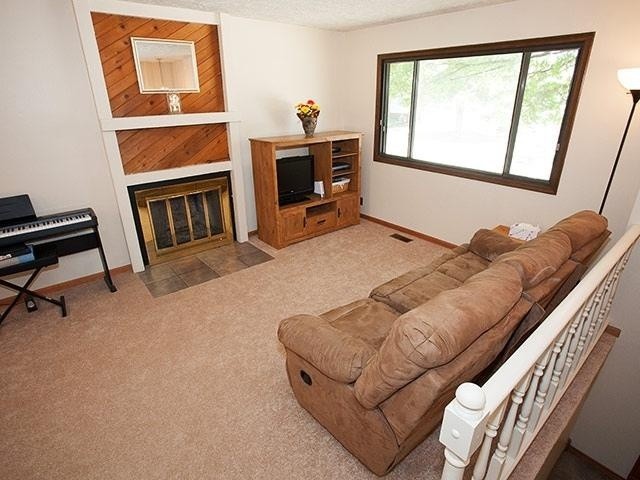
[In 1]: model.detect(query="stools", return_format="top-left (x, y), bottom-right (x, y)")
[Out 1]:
top-left (1, 244), bottom-right (68, 324)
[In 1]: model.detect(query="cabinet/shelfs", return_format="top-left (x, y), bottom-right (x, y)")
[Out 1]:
top-left (248, 130), bottom-right (363, 250)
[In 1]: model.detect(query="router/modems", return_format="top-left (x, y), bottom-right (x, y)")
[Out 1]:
top-left (314, 180), bottom-right (325, 198)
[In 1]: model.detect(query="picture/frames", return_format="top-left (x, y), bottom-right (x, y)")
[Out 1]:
top-left (130, 36), bottom-right (201, 94)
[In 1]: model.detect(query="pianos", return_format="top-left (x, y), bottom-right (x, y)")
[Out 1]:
top-left (0, 208), bottom-right (117, 324)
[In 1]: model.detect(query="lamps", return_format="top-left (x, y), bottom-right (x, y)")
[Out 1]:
top-left (599, 67), bottom-right (640, 216)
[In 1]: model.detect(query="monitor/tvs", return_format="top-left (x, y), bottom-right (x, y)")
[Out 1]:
top-left (276, 155), bottom-right (315, 207)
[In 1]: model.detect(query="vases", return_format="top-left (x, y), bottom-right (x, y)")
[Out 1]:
top-left (303, 117), bottom-right (317, 138)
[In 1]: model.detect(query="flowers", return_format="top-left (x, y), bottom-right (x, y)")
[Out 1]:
top-left (295, 100), bottom-right (319, 118)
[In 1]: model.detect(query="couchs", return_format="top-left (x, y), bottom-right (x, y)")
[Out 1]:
top-left (277, 208), bottom-right (611, 477)
